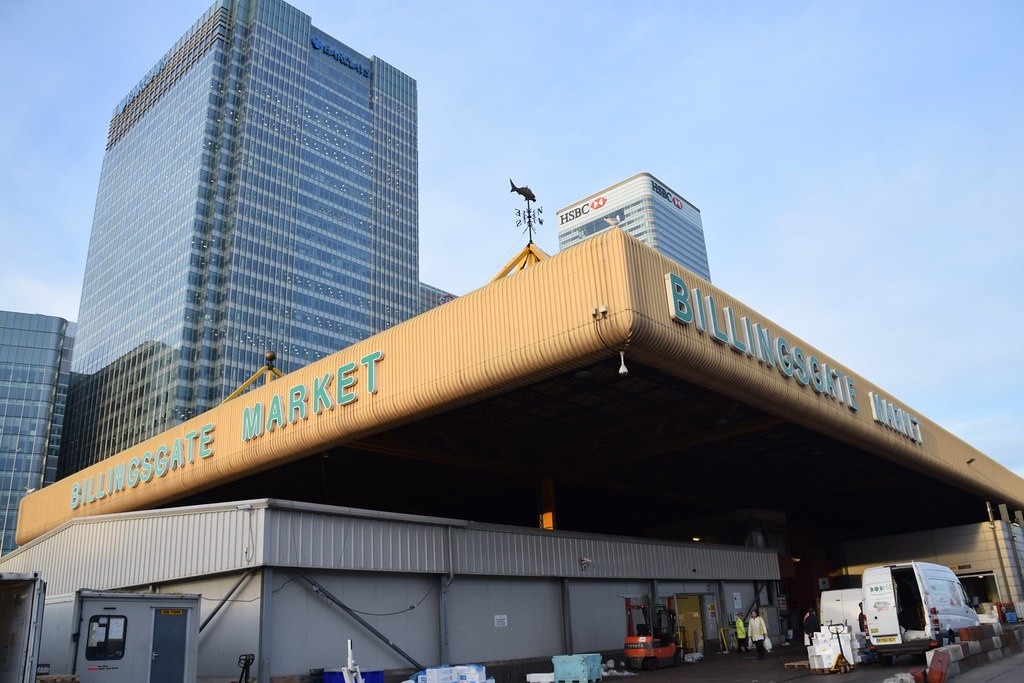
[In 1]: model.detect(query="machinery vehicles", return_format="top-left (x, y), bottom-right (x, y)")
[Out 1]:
top-left (625, 604), bottom-right (685, 672)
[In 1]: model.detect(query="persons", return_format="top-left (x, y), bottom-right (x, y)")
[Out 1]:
top-left (804, 607), bottom-right (817, 637)
top-left (736, 613), bottom-right (750, 653)
top-left (748, 610), bottom-right (769, 660)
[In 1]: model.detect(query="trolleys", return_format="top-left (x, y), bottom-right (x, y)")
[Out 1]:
top-left (828, 623), bottom-right (856, 674)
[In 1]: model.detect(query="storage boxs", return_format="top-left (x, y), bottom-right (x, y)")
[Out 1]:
top-left (419, 664), bottom-right (496, 683)
top-left (804, 617), bottom-right (868, 670)
top-left (526, 672), bottom-right (555, 683)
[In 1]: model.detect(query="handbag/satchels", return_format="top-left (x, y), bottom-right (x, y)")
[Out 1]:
top-left (764, 636), bottom-right (772, 653)
top-left (747, 638), bottom-right (753, 650)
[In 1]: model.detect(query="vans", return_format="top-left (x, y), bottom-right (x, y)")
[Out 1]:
top-left (820, 587), bottom-right (862, 637)
top-left (862, 561), bottom-right (981, 666)
